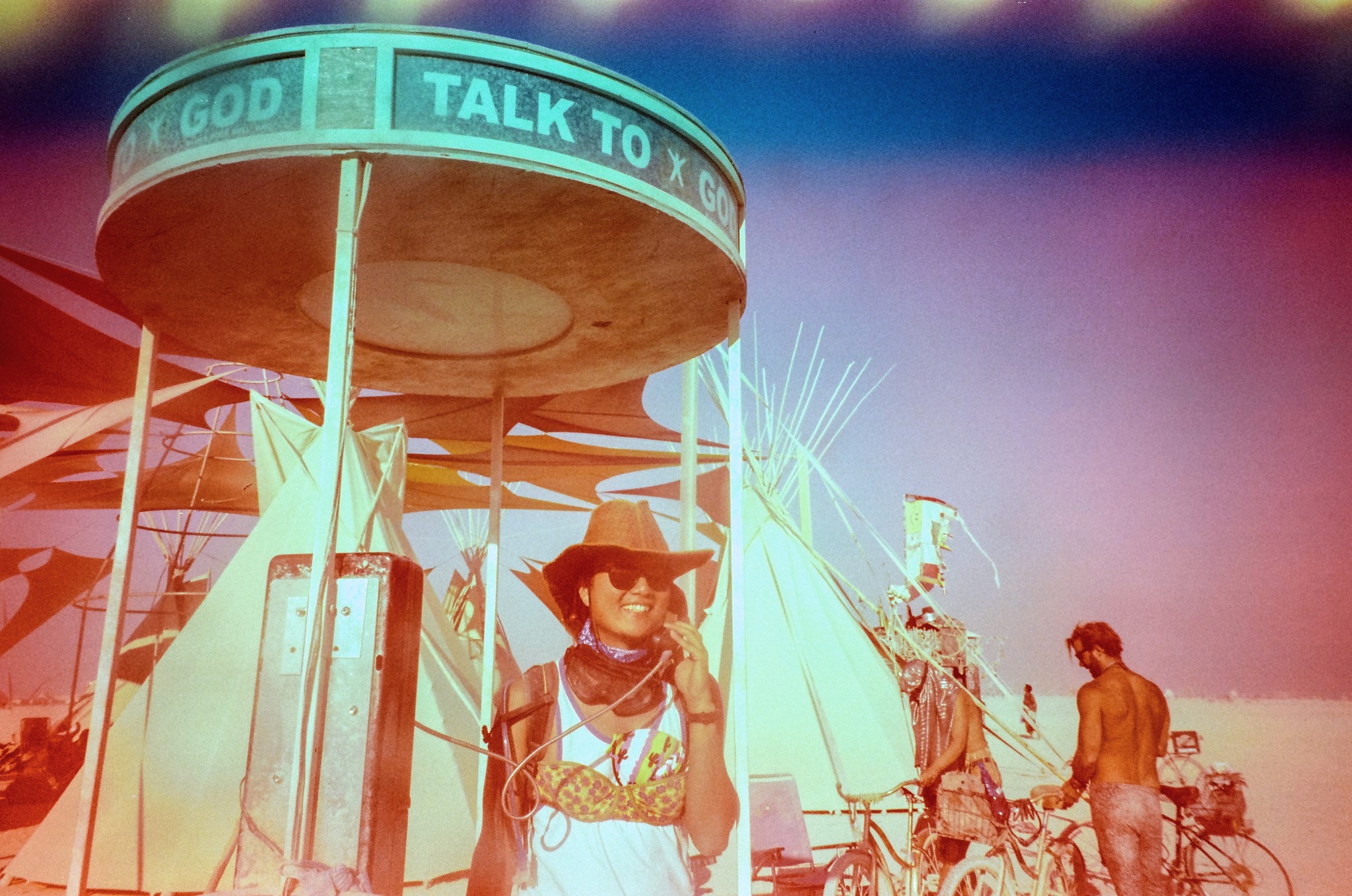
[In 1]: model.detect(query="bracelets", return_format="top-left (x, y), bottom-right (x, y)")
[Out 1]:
top-left (1070, 777), bottom-right (1087, 789)
top-left (684, 710), bottom-right (724, 725)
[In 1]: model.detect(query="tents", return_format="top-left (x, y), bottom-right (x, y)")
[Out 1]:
top-left (652, 490), bottom-right (924, 896)
top-left (14, 406), bottom-right (511, 896)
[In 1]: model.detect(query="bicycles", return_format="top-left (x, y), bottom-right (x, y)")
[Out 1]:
top-left (1053, 786), bottom-right (1293, 896)
top-left (942, 785), bottom-right (1091, 895)
top-left (825, 779), bottom-right (976, 895)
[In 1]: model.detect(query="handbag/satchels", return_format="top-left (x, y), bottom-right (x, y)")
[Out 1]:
top-left (934, 770), bottom-right (1008, 847)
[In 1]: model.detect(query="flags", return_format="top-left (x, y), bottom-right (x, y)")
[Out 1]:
top-left (888, 493), bottom-right (959, 606)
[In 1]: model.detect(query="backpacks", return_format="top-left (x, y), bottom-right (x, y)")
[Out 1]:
top-left (1198, 771), bottom-right (1246, 834)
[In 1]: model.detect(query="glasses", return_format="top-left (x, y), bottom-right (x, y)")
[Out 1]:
top-left (1075, 648), bottom-right (1087, 661)
top-left (564, 643), bottom-right (666, 717)
top-left (583, 560), bottom-right (677, 591)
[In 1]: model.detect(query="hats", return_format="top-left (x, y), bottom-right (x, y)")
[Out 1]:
top-left (541, 499), bottom-right (716, 640)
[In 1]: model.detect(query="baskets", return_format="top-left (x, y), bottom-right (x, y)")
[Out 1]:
top-left (932, 787), bottom-right (1009, 849)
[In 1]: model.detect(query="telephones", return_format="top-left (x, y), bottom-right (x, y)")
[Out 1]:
top-left (651, 584), bottom-right (690, 657)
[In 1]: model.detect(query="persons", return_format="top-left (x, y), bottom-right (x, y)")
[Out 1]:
top-left (1020, 685), bottom-right (1042, 740)
top-left (1062, 622), bottom-right (1170, 896)
top-left (919, 632), bottom-right (1002, 896)
top-left (467, 500), bottom-right (738, 896)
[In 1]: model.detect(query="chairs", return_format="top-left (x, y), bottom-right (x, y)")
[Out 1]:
top-left (749, 772), bottom-right (857, 896)
